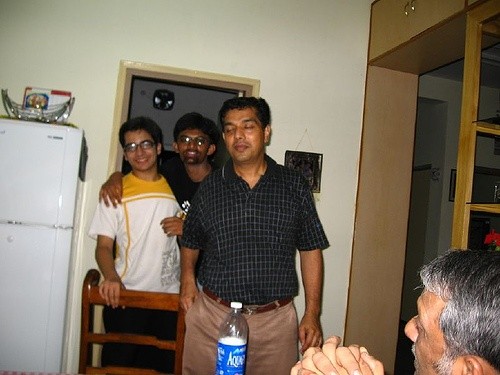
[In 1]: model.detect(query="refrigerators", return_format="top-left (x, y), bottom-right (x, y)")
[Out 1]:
top-left (0, 115), bottom-right (84, 375)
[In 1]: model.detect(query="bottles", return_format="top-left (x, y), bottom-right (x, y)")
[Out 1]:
top-left (215, 302), bottom-right (249, 375)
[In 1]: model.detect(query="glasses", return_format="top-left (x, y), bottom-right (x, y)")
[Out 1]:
top-left (175, 132), bottom-right (215, 148)
top-left (124, 137), bottom-right (158, 154)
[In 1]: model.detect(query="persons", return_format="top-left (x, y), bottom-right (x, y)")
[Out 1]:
top-left (97, 111), bottom-right (226, 289)
top-left (289, 248), bottom-right (500, 375)
top-left (180, 97), bottom-right (330, 374)
top-left (87, 116), bottom-right (188, 375)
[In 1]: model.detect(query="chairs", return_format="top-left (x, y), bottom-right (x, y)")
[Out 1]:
top-left (77, 268), bottom-right (185, 375)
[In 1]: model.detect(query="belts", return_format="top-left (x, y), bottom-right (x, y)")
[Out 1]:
top-left (200, 283), bottom-right (294, 315)
